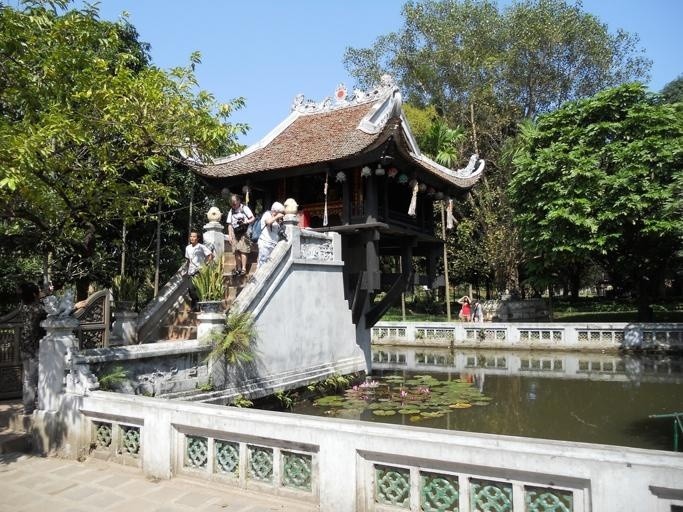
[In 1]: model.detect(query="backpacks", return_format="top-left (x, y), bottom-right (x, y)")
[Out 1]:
top-left (249, 216), bottom-right (268, 243)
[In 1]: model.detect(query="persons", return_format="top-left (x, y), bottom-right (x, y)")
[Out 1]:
top-left (474, 371), bottom-right (484, 393)
top-left (20, 282), bottom-right (87, 415)
top-left (473, 302), bottom-right (484, 321)
top-left (457, 295), bottom-right (471, 323)
top-left (225, 195), bottom-right (255, 276)
top-left (183, 230), bottom-right (214, 313)
top-left (458, 373), bottom-right (474, 387)
top-left (256, 202), bottom-right (285, 268)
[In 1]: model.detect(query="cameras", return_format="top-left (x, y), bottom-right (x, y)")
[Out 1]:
top-left (278, 229), bottom-right (287, 239)
top-left (237, 218), bottom-right (243, 224)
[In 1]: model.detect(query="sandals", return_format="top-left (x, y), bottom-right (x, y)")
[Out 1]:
top-left (232, 270), bottom-right (247, 277)
